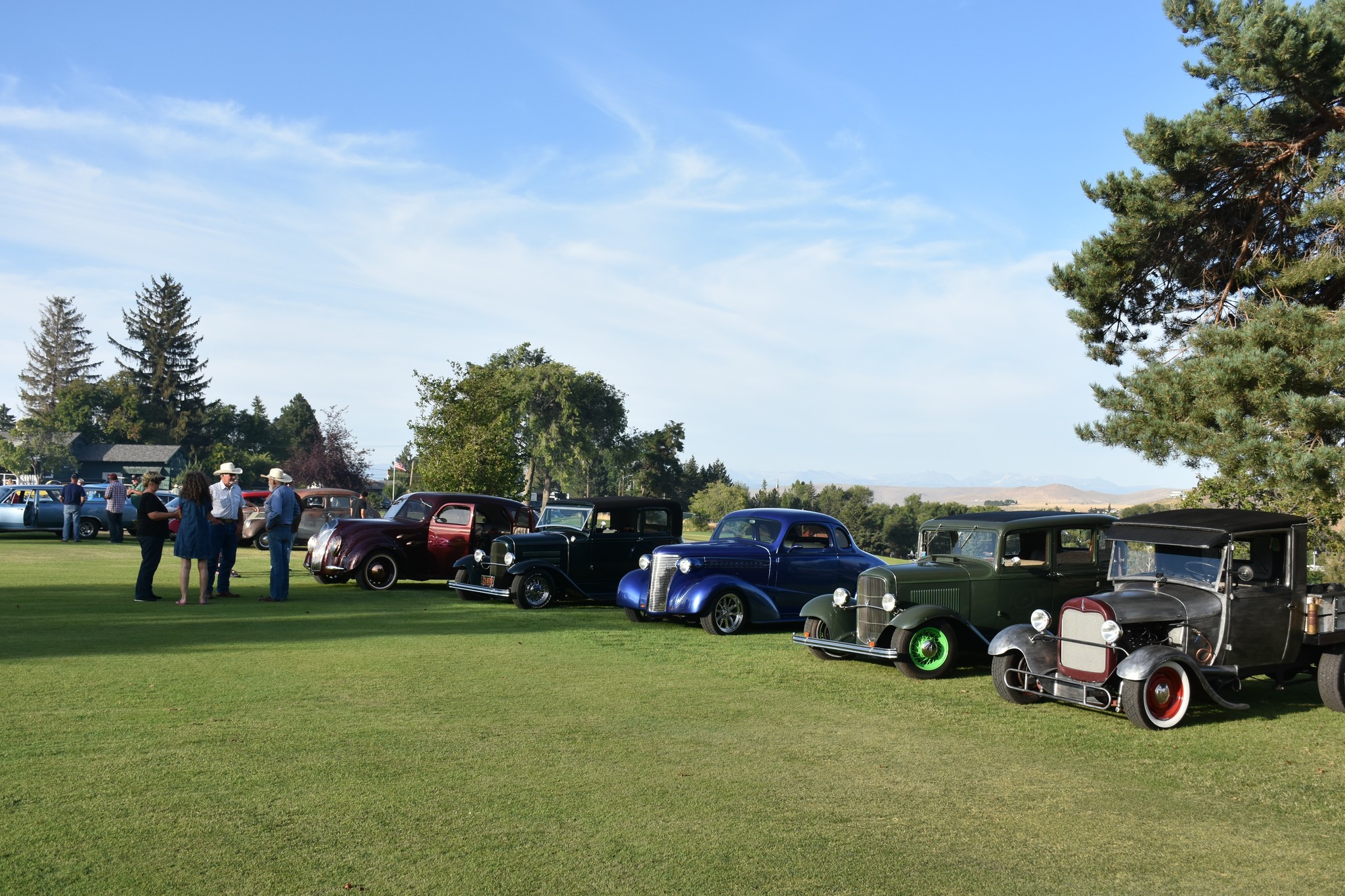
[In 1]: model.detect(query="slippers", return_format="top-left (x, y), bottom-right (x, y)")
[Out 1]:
top-left (175, 600), bottom-right (188, 605)
top-left (199, 600), bottom-right (208, 604)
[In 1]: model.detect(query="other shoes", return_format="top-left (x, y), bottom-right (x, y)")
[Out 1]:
top-left (74, 540), bottom-right (82, 542)
top-left (258, 595), bottom-right (287, 602)
top-left (134, 593), bottom-right (162, 602)
top-left (215, 565), bottom-right (241, 578)
top-left (62, 540), bottom-right (68, 542)
top-left (205, 592), bottom-right (215, 599)
top-left (216, 590), bottom-right (241, 598)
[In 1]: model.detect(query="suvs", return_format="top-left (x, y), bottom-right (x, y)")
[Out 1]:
top-left (790, 513), bottom-right (1129, 680)
top-left (444, 496), bottom-right (687, 610)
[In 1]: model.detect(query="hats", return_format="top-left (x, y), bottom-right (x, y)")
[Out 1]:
top-left (260, 468), bottom-right (293, 483)
top-left (71, 473), bottom-right (80, 479)
top-left (174, 484), bottom-right (179, 487)
top-left (131, 474), bottom-right (139, 480)
top-left (213, 462), bottom-right (243, 476)
top-left (0, 478), bottom-right (2, 480)
top-left (108, 473), bottom-right (117, 479)
top-left (148, 476), bottom-right (166, 482)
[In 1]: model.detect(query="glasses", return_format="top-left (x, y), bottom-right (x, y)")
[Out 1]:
top-left (153, 480), bottom-right (162, 484)
top-left (131, 479), bottom-right (135, 481)
top-left (80, 482), bottom-right (84, 484)
top-left (224, 473), bottom-right (236, 477)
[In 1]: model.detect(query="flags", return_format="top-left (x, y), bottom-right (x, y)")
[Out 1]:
top-left (395, 457), bottom-right (406, 471)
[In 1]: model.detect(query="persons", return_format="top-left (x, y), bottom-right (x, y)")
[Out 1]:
top-left (6, 478), bottom-right (14, 485)
top-left (265, 482), bottom-right (306, 571)
top-left (172, 484), bottom-right (181, 494)
top-left (59, 474), bottom-right (86, 543)
top-left (104, 474), bottom-right (127, 543)
top-left (175, 472), bottom-right (213, 605)
top-left (350, 491), bottom-right (369, 518)
top-left (258, 468), bottom-right (300, 602)
top-left (127, 474), bottom-right (146, 519)
top-left (206, 462), bottom-right (246, 599)
top-left (0, 478), bottom-right (3, 494)
top-left (76, 478), bottom-right (88, 501)
top-left (216, 475), bottom-right (241, 578)
top-left (134, 470), bottom-right (180, 601)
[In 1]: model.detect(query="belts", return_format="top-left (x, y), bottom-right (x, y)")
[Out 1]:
top-left (276, 523), bottom-right (291, 526)
top-left (65, 503), bottom-right (80, 505)
top-left (216, 517), bottom-right (239, 524)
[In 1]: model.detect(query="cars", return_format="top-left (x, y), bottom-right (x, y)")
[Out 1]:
top-left (167, 490), bottom-right (270, 541)
top-left (303, 491), bottom-right (541, 591)
top-left (239, 488), bottom-right (381, 551)
top-left (155, 492), bottom-right (185, 524)
top-left (0, 485), bottom-right (138, 540)
top-left (616, 508), bottom-right (891, 636)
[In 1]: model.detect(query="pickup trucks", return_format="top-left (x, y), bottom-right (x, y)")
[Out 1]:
top-left (987, 508), bottom-right (1345, 730)
